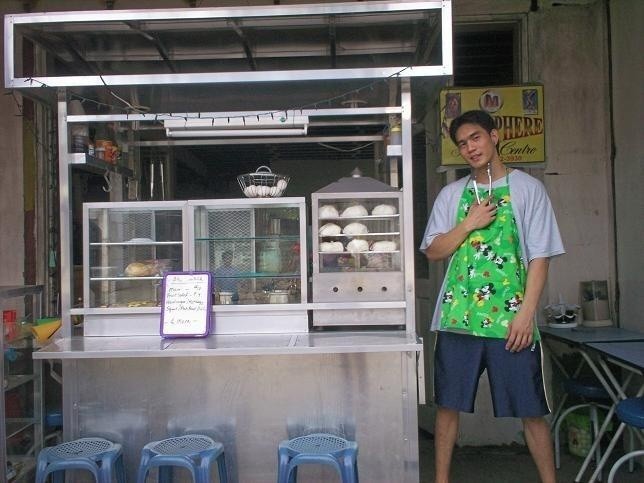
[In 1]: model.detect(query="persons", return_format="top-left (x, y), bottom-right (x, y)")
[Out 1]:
top-left (212, 250), bottom-right (245, 303)
top-left (418, 108), bottom-right (567, 482)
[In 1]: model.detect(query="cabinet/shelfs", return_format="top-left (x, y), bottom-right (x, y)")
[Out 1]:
top-left (311, 192), bottom-right (404, 327)
top-left (85, 201), bottom-right (187, 336)
top-left (187, 199), bottom-right (308, 334)
top-left (0, 286), bottom-right (45, 483)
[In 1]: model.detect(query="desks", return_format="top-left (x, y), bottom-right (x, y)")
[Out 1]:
top-left (539, 326), bottom-right (644, 431)
top-left (573, 339), bottom-right (644, 483)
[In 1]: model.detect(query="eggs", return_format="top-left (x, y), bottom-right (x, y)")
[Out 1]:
top-left (243, 178), bottom-right (287, 197)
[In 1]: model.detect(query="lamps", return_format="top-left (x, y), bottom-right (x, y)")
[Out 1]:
top-left (164, 116), bottom-right (310, 137)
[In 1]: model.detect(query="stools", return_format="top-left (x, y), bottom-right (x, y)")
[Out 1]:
top-left (137, 434), bottom-right (227, 483)
top-left (278, 434), bottom-right (359, 483)
top-left (554, 378), bottom-right (609, 482)
top-left (35, 437), bottom-right (127, 483)
top-left (608, 396), bottom-right (644, 483)
